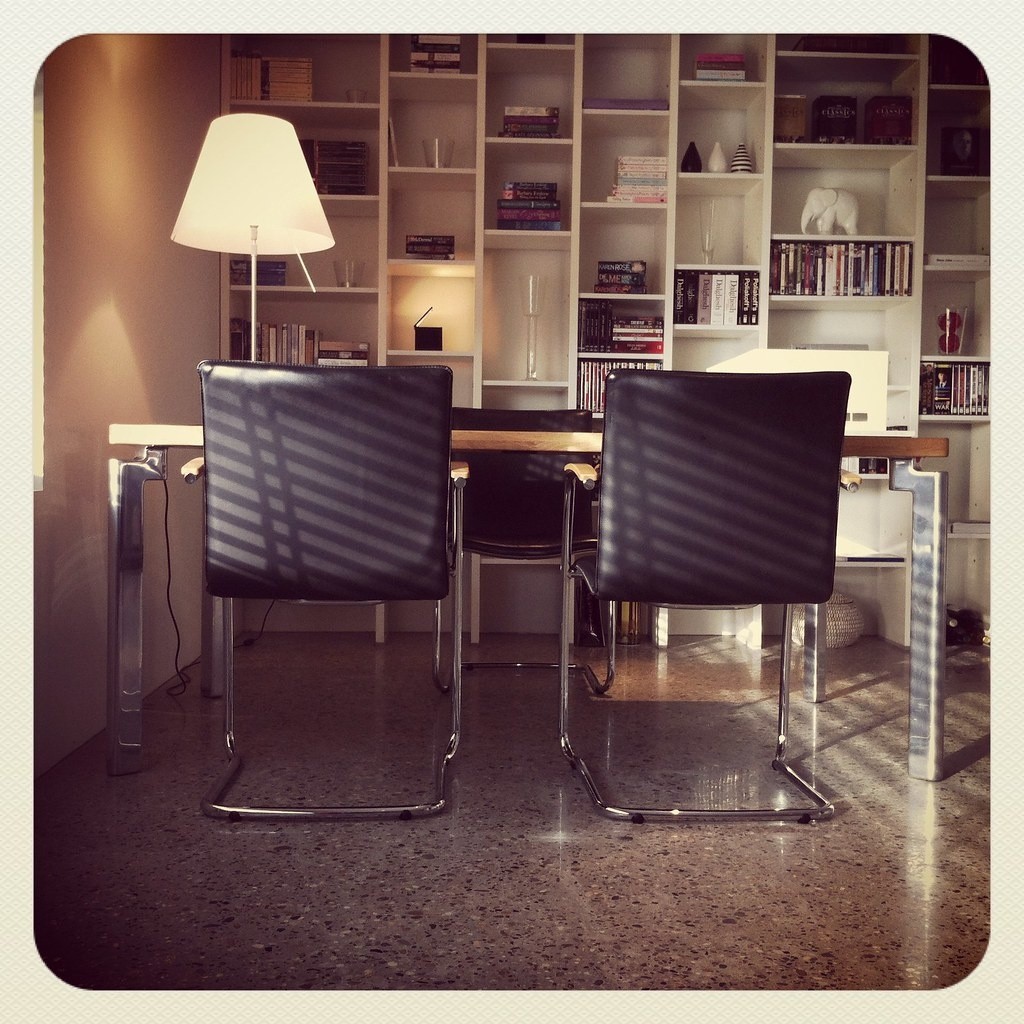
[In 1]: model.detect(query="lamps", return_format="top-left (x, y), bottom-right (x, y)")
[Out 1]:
top-left (171, 113), bottom-right (338, 359)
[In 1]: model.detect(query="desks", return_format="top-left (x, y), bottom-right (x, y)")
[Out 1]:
top-left (99, 423), bottom-right (954, 782)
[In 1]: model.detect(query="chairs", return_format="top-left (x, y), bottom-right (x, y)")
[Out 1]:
top-left (558, 368), bottom-right (853, 823)
top-left (195, 357), bottom-right (471, 818)
top-left (434, 408), bottom-right (617, 692)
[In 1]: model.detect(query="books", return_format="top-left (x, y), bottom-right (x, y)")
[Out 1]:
top-left (577, 361), bottom-right (662, 412)
top-left (232, 56), bottom-right (316, 101)
top-left (299, 139), bottom-right (374, 195)
top-left (606, 156), bottom-right (667, 203)
top-left (693, 53), bottom-right (747, 81)
top-left (865, 96), bottom-right (913, 144)
top-left (594, 261), bottom-right (648, 294)
top-left (578, 301), bottom-right (664, 354)
top-left (812, 95), bottom-right (858, 144)
top-left (582, 98), bottom-right (668, 110)
top-left (409, 35), bottom-right (461, 74)
top-left (773, 94), bottom-right (808, 143)
top-left (230, 317), bottom-right (370, 366)
top-left (231, 260), bottom-right (290, 285)
top-left (405, 234), bottom-right (455, 260)
top-left (768, 243), bottom-right (911, 296)
top-left (940, 126), bottom-right (980, 176)
top-left (792, 35), bottom-right (907, 54)
top-left (498, 105), bottom-right (562, 138)
top-left (920, 362), bottom-right (989, 416)
top-left (497, 181), bottom-right (561, 231)
top-left (673, 271), bottom-right (759, 326)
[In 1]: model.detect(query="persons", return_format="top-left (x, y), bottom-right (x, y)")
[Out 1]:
top-left (938, 371), bottom-right (948, 387)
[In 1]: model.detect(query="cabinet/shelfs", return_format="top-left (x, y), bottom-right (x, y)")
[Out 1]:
top-left (222, 36), bottom-right (990, 654)
